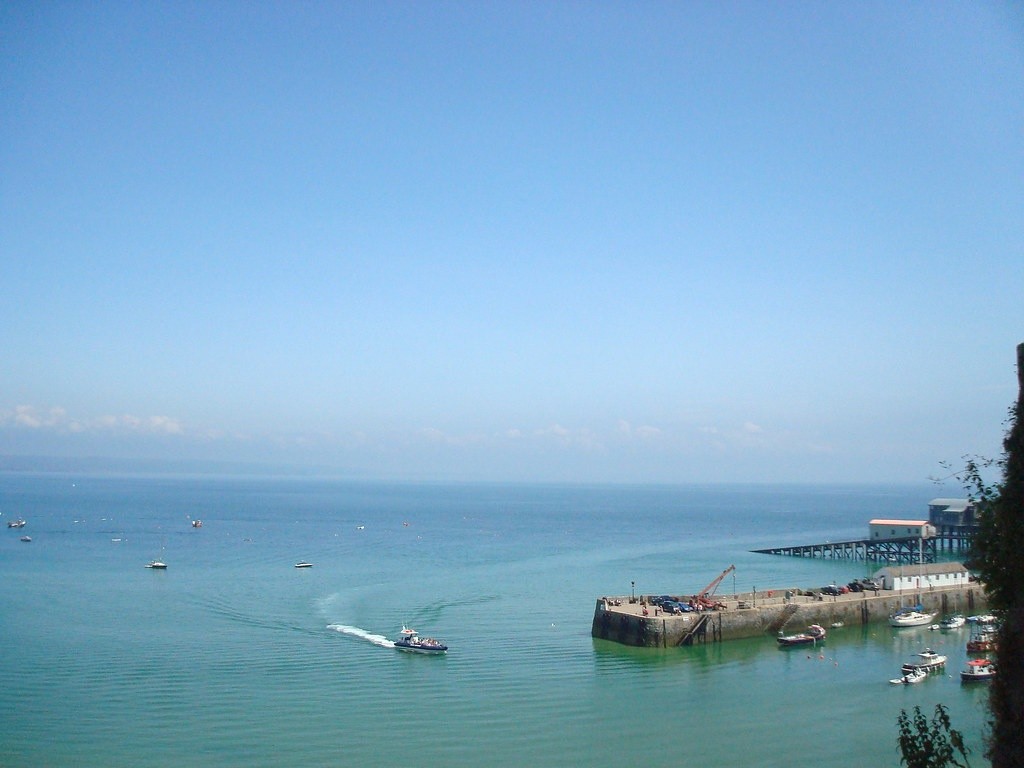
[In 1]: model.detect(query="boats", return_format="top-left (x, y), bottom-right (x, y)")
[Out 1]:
top-left (965, 612), bottom-right (997, 633)
top-left (294, 562), bottom-right (313, 567)
top-left (940, 614), bottom-right (965, 629)
top-left (356, 525), bottom-right (365, 529)
top-left (145, 558), bottom-right (168, 568)
top-left (889, 611), bottom-right (937, 626)
top-left (961, 659), bottom-right (997, 681)
top-left (20, 536), bottom-right (32, 542)
top-left (901, 647), bottom-right (947, 673)
top-left (831, 620), bottom-right (845, 627)
top-left (400, 621), bottom-right (418, 634)
top-left (966, 621), bottom-right (998, 652)
top-left (393, 621), bottom-right (448, 655)
top-left (890, 668), bottom-right (926, 684)
top-left (928, 624), bottom-right (938, 630)
top-left (402, 521), bottom-right (408, 526)
top-left (777, 625), bottom-right (825, 645)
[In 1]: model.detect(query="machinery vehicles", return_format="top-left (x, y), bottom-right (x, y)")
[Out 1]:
top-left (690, 565), bottom-right (738, 611)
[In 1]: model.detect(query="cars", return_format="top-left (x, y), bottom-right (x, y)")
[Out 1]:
top-left (648, 595), bottom-right (694, 613)
top-left (859, 578), bottom-right (883, 590)
top-left (820, 585), bottom-right (841, 595)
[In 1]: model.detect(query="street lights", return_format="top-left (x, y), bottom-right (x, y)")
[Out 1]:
top-left (733, 572), bottom-right (736, 595)
top-left (631, 581), bottom-right (635, 599)
top-left (753, 585), bottom-right (756, 608)
